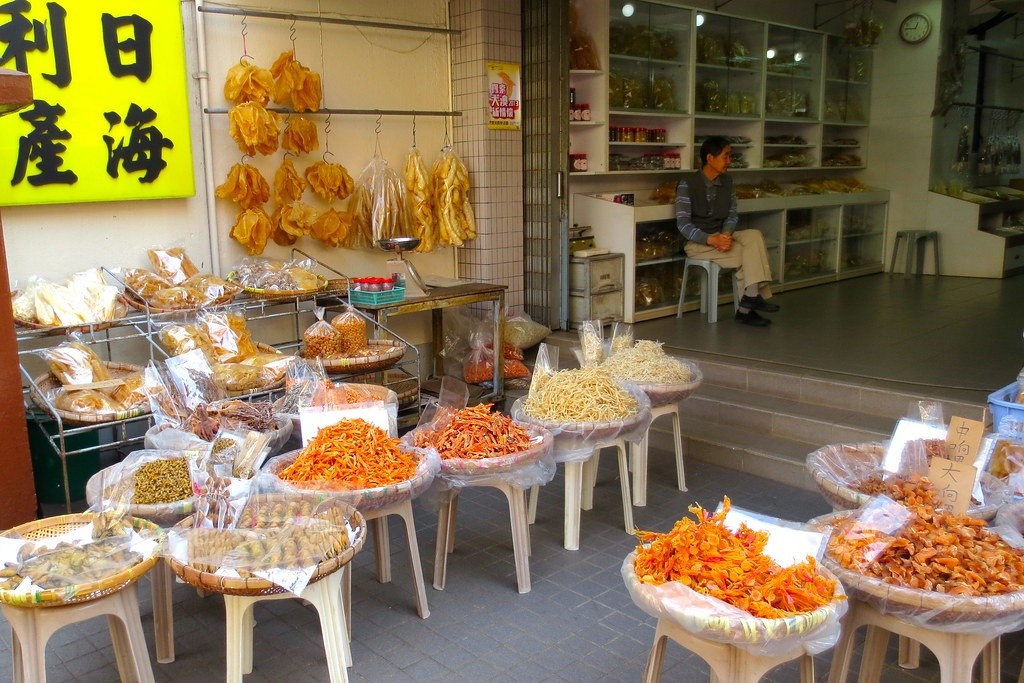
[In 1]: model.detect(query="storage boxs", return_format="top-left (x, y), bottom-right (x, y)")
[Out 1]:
top-left (350, 286), bottom-right (406, 305)
top-left (988, 380), bottom-right (1024, 443)
top-left (26, 405), bottom-right (101, 503)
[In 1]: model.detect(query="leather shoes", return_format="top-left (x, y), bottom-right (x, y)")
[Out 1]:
top-left (739, 294), bottom-right (780, 313)
top-left (734, 309), bottom-right (771, 327)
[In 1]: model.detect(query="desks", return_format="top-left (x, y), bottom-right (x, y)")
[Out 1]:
top-left (316, 277), bottom-right (508, 432)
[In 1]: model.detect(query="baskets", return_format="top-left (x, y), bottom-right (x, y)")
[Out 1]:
top-left (6, 232), bottom-right (371, 336)
top-left (619, 438), bottom-right (1024, 647)
top-left (350, 286), bottom-right (405, 306)
top-left (0, 353), bottom-right (704, 607)
top-left (29, 336), bottom-right (421, 426)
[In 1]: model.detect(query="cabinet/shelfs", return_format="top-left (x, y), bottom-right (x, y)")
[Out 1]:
top-left (568, 0), bottom-right (876, 175)
top-left (574, 180), bottom-right (890, 324)
top-left (17, 247), bottom-right (422, 514)
top-left (929, 184), bottom-right (1024, 279)
top-left (569, 248), bottom-right (624, 329)
top-left (197, 0), bottom-right (466, 119)
top-left (955, 45), bottom-right (1024, 153)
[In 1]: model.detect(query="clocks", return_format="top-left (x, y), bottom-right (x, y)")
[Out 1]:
top-left (899, 12), bottom-right (931, 44)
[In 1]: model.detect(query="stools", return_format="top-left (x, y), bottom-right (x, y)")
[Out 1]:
top-left (891, 229), bottom-right (940, 281)
top-left (132, 523), bottom-right (257, 664)
top-left (222, 566), bottom-right (349, 683)
top-left (432, 471), bottom-right (532, 594)
top-left (303, 499), bottom-right (430, 644)
top-left (677, 258), bottom-right (741, 323)
top-left (592, 402), bottom-right (688, 507)
top-left (827, 588), bottom-right (1000, 683)
top-left (644, 619), bottom-right (815, 682)
top-left (0, 581), bottom-right (156, 683)
top-left (528, 437), bottom-right (638, 551)
top-left (832, 504), bottom-right (922, 670)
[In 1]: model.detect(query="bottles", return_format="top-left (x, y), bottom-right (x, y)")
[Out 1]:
top-left (570, 88), bottom-right (576, 104)
top-left (610, 126), bottom-right (666, 142)
top-left (569, 104), bottom-right (591, 121)
top-left (353, 277), bottom-right (395, 292)
top-left (570, 154), bottom-right (587, 172)
top-left (644, 153), bottom-right (681, 169)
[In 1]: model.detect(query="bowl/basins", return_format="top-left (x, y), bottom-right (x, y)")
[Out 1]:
top-left (376, 238), bottom-right (420, 251)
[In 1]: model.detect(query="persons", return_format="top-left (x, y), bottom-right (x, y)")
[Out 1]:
top-left (675, 135), bottom-right (781, 327)
